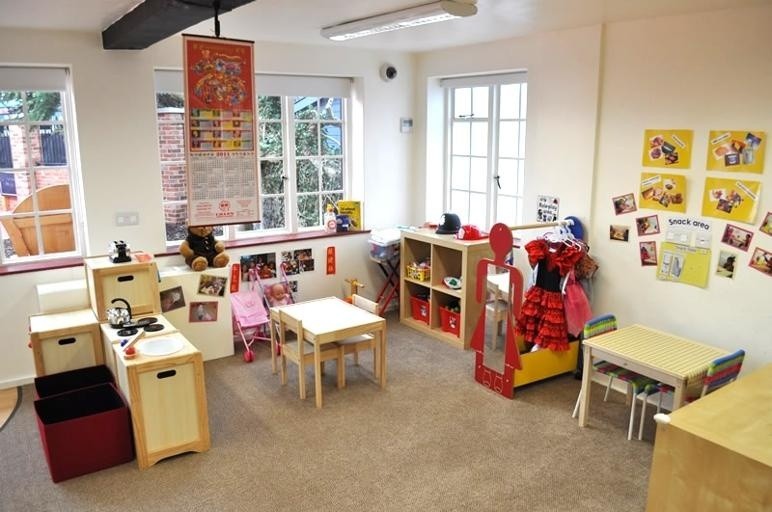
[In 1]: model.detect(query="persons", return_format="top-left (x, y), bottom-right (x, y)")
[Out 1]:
top-left (269, 284), bottom-right (293, 308)
top-left (196, 304), bottom-right (206, 321)
top-left (242, 256), bottom-right (276, 281)
top-left (610, 135), bottom-right (772, 278)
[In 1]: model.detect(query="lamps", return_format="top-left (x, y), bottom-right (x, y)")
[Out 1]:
top-left (320, 2), bottom-right (478, 44)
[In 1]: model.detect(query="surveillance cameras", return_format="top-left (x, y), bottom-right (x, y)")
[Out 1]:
top-left (381, 63), bottom-right (397, 82)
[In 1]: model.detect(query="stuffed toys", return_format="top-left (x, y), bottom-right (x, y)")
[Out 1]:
top-left (180, 220), bottom-right (229, 271)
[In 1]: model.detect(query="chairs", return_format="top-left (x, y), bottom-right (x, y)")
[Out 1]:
top-left (278, 311), bottom-right (342, 400)
top-left (639, 350), bottom-right (748, 440)
top-left (485, 277), bottom-right (508, 351)
top-left (572, 312), bottom-right (657, 440)
top-left (335, 295), bottom-right (380, 386)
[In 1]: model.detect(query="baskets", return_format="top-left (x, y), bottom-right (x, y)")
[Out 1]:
top-left (406, 263), bottom-right (431, 281)
top-left (411, 294), bottom-right (429, 325)
top-left (440, 304), bottom-right (461, 336)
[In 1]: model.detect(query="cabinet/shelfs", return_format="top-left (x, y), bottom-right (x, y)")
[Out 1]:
top-left (400, 228), bottom-right (495, 350)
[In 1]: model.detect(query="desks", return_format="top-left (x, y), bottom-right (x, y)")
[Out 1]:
top-left (489, 272), bottom-right (513, 304)
top-left (372, 256), bottom-right (403, 317)
top-left (576, 324), bottom-right (735, 428)
top-left (268, 295), bottom-right (388, 409)
top-left (649, 361), bottom-right (772, 512)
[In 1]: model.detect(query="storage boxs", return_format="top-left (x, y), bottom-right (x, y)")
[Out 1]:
top-left (407, 259), bottom-right (432, 285)
top-left (437, 301), bottom-right (461, 337)
top-left (368, 238), bottom-right (403, 261)
top-left (408, 291), bottom-right (431, 325)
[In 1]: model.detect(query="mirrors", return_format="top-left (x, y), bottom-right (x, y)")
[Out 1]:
top-left (469, 223), bottom-right (523, 399)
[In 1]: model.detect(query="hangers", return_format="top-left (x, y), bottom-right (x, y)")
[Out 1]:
top-left (546, 217), bottom-right (580, 247)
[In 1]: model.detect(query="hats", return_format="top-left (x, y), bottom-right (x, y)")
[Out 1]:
top-left (436, 214), bottom-right (460, 234)
top-left (457, 226), bottom-right (489, 240)
